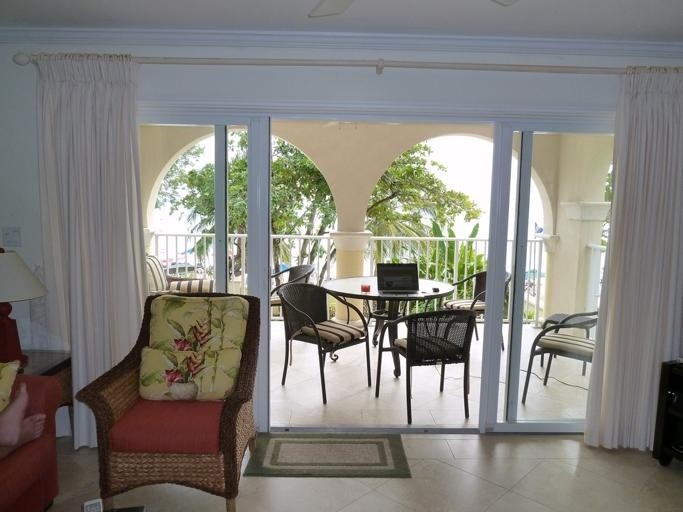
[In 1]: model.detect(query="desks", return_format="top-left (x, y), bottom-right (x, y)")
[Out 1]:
top-left (18, 349), bottom-right (74, 441)
top-left (323, 277), bottom-right (454, 378)
top-left (651, 360), bottom-right (683, 467)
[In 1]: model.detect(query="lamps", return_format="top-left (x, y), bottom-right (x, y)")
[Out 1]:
top-left (0, 248), bottom-right (50, 368)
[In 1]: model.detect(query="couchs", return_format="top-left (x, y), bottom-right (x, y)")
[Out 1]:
top-left (0, 373), bottom-right (62, 512)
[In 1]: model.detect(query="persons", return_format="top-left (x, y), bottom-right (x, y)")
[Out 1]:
top-left (1, 381), bottom-right (49, 458)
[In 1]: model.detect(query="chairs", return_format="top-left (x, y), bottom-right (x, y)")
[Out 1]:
top-left (278, 282), bottom-right (371, 404)
top-left (540, 313), bottom-right (597, 376)
top-left (521, 311), bottom-right (598, 404)
top-left (75, 293), bottom-right (261, 512)
top-left (271, 265), bottom-right (315, 366)
top-left (443, 270), bottom-right (511, 351)
top-left (145, 256), bottom-right (214, 295)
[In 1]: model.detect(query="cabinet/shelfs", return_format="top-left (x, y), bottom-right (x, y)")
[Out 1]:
top-left (375, 309), bottom-right (523, 425)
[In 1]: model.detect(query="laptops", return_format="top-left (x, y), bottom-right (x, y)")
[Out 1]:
top-left (377, 263), bottom-right (419, 294)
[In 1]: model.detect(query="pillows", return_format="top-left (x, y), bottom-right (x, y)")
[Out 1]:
top-left (0, 360), bottom-right (20, 412)
top-left (138, 345), bottom-right (218, 402)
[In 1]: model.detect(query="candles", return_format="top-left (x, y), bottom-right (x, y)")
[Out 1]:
top-left (361, 285), bottom-right (370, 292)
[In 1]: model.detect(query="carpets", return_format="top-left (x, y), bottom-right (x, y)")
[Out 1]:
top-left (243, 432), bottom-right (412, 478)
